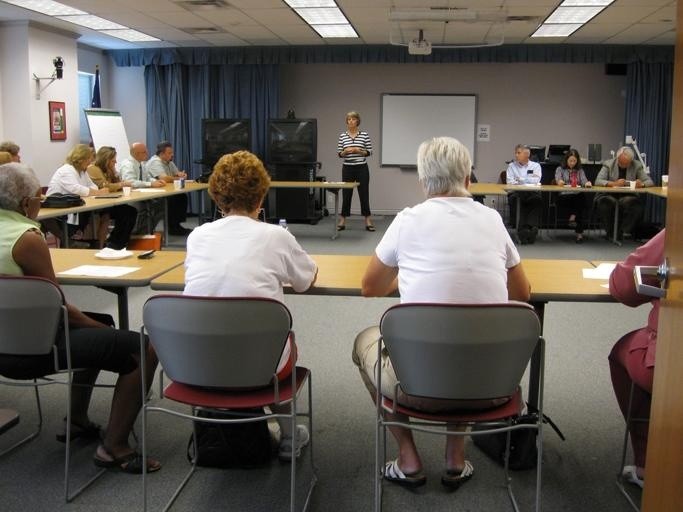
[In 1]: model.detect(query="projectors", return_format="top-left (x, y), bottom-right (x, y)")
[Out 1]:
top-left (409, 39), bottom-right (431, 55)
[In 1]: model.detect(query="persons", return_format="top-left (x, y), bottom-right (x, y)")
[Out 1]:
top-left (335, 110), bottom-right (379, 233)
top-left (593, 146), bottom-right (654, 241)
top-left (506, 144), bottom-right (544, 246)
top-left (0, 161), bottom-right (164, 475)
top-left (0, 141), bottom-right (192, 250)
top-left (606, 226), bottom-right (667, 490)
top-left (350, 136), bottom-right (531, 486)
top-left (182, 150), bottom-right (319, 465)
top-left (554, 148), bottom-right (592, 244)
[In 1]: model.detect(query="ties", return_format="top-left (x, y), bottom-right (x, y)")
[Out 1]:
top-left (139, 164), bottom-right (142, 180)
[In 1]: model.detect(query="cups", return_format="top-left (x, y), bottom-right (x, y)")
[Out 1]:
top-left (173, 179), bottom-right (180, 188)
top-left (122, 186), bottom-right (131, 197)
top-left (661, 175), bottom-right (667, 189)
top-left (629, 181), bottom-right (636, 190)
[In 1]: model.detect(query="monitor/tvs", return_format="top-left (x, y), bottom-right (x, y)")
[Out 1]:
top-left (201, 119), bottom-right (252, 160)
top-left (267, 118), bottom-right (317, 162)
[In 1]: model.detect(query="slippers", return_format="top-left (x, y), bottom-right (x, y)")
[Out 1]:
top-left (622, 466), bottom-right (644, 489)
top-left (568, 219), bottom-right (576, 225)
top-left (441, 460), bottom-right (473, 486)
top-left (576, 237), bottom-right (584, 244)
top-left (366, 226), bottom-right (375, 231)
top-left (337, 225), bottom-right (345, 230)
top-left (380, 458), bottom-right (426, 487)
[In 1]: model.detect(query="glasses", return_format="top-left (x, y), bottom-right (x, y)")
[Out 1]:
top-left (32, 194), bottom-right (47, 202)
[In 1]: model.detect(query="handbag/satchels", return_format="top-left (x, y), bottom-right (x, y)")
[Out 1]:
top-left (472, 417), bottom-right (538, 471)
top-left (187, 408), bottom-right (271, 469)
top-left (41, 193), bottom-right (83, 207)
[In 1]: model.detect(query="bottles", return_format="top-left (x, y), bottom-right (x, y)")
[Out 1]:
top-left (179, 177), bottom-right (185, 189)
top-left (570, 170), bottom-right (576, 187)
top-left (278, 218), bottom-right (290, 233)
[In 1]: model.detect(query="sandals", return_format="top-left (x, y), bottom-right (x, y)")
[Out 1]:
top-left (93, 444), bottom-right (161, 473)
top-left (55, 417), bottom-right (102, 442)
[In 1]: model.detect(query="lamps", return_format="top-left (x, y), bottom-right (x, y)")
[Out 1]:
top-left (32, 55), bottom-right (65, 100)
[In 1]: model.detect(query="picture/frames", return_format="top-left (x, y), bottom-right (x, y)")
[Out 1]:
top-left (49, 101), bottom-right (67, 140)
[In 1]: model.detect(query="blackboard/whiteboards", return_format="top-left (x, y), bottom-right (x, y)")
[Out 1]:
top-left (378, 93), bottom-right (478, 168)
top-left (84, 108), bottom-right (133, 173)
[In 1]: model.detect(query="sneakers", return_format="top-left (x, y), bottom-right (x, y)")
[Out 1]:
top-left (519, 226), bottom-right (538, 244)
top-left (279, 425), bottom-right (309, 460)
